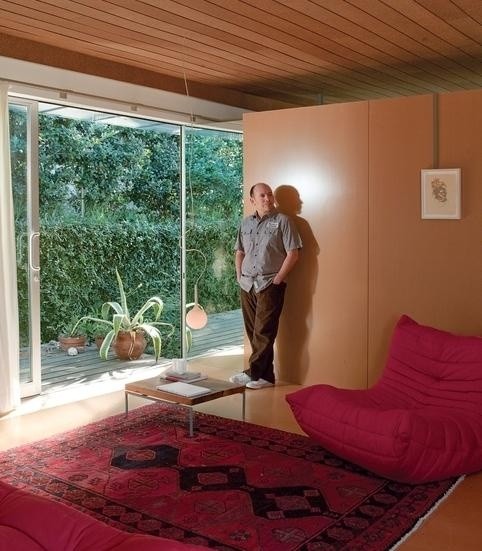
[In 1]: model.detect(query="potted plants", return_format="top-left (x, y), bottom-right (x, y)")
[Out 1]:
top-left (70, 267), bottom-right (175, 361)
top-left (58, 315), bottom-right (89, 352)
top-left (89, 306), bottom-right (113, 350)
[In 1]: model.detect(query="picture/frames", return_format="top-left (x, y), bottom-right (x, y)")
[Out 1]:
top-left (421, 168), bottom-right (461, 220)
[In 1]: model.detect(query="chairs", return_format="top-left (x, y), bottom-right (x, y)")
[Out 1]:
top-left (286, 315), bottom-right (482, 483)
top-left (0, 481), bottom-right (212, 551)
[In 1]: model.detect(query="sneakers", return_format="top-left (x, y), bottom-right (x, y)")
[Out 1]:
top-left (229, 373), bottom-right (251, 385)
top-left (246, 378), bottom-right (273, 389)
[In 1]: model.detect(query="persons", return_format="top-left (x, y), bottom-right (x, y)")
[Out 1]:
top-left (228, 183), bottom-right (303, 390)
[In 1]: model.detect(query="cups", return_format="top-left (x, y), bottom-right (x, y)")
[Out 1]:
top-left (172, 358), bottom-right (187, 375)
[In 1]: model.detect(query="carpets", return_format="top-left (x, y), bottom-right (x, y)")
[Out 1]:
top-left (0, 401), bottom-right (464, 551)
